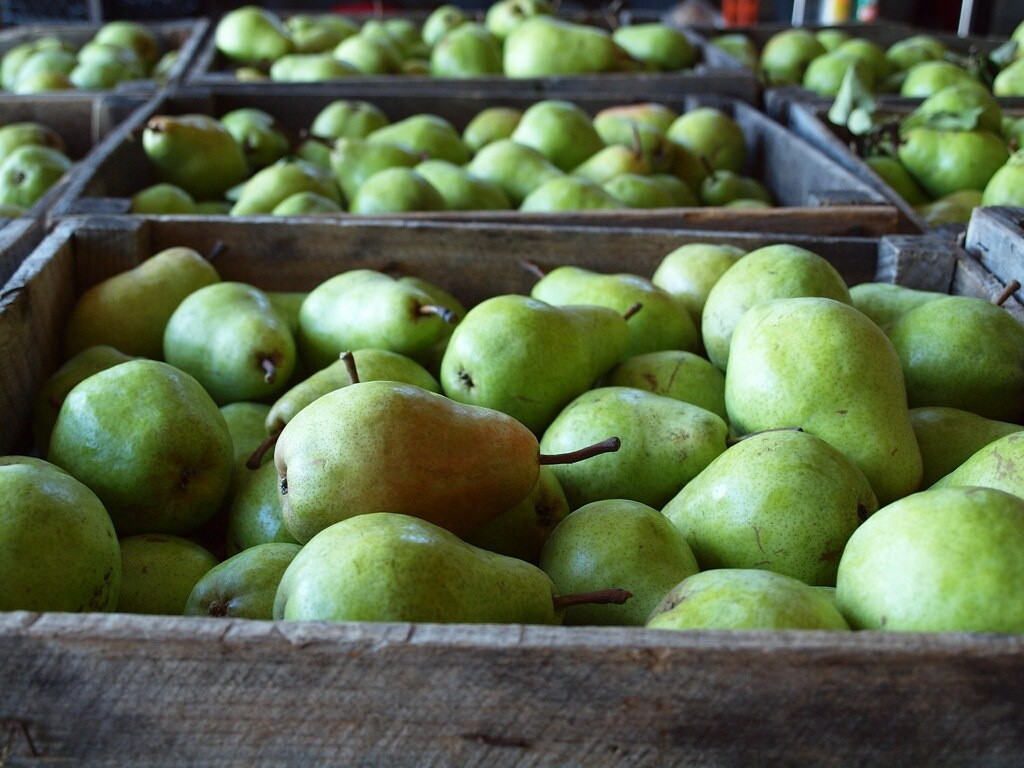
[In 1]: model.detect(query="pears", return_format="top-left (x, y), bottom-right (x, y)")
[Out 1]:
top-left (0, 0), bottom-right (1024, 639)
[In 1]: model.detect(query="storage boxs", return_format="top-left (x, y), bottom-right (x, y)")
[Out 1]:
top-left (0, 12), bottom-right (1024, 768)
